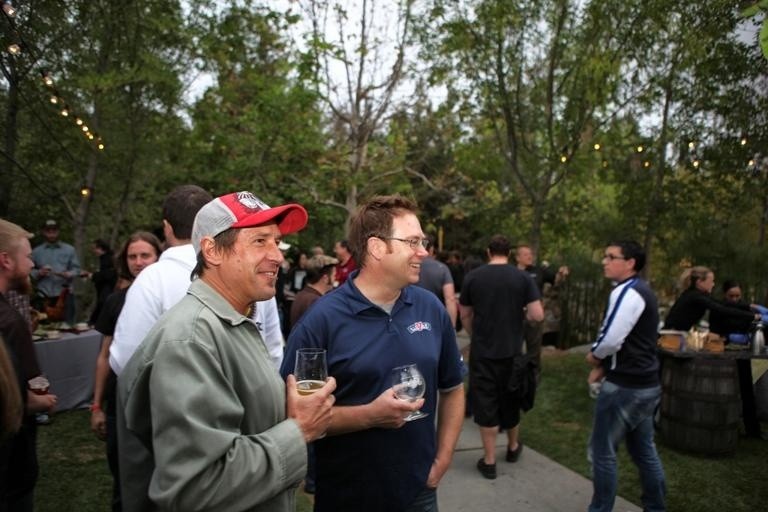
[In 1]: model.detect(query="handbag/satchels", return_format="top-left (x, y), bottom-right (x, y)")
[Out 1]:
top-left (510, 352), bottom-right (538, 414)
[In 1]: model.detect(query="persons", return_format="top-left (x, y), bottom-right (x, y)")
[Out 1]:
top-left (90, 183), bottom-right (286, 510)
top-left (413, 234), bottom-right (568, 480)
top-left (281, 189), bottom-right (468, 512)
top-left (663, 265), bottom-right (768, 344)
top-left (0, 219), bottom-right (58, 511)
top-left (31, 218), bottom-right (117, 324)
top-left (274, 241), bottom-right (357, 342)
top-left (118, 191), bottom-right (337, 511)
top-left (586, 241), bottom-right (669, 510)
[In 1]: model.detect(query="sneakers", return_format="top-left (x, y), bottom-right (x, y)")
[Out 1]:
top-left (506, 440), bottom-right (523, 463)
top-left (477, 457), bottom-right (497, 479)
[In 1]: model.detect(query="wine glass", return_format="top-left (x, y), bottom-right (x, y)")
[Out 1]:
top-left (391, 362), bottom-right (430, 422)
top-left (293, 348), bottom-right (329, 440)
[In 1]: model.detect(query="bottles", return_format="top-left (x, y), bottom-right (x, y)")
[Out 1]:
top-left (29, 375), bottom-right (52, 425)
top-left (751, 323), bottom-right (766, 357)
top-left (697, 321), bottom-right (709, 344)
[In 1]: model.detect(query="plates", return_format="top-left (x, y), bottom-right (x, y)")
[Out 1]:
top-left (30, 325), bottom-right (90, 340)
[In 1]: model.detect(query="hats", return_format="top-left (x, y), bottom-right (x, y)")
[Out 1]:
top-left (191, 190), bottom-right (310, 258)
top-left (43, 219), bottom-right (61, 230)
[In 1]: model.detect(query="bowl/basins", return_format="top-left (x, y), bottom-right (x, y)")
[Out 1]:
top-left (730, 333), bottom-right (748, 345)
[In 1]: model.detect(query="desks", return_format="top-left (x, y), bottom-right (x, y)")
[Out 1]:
top-left (659, 332), bottom-right (754, 457)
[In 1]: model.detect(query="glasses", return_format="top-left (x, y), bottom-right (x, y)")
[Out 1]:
top-left (603, 253), bottom-right (627, 261)
top-left (368, 235), bottom-right (430, 250)
